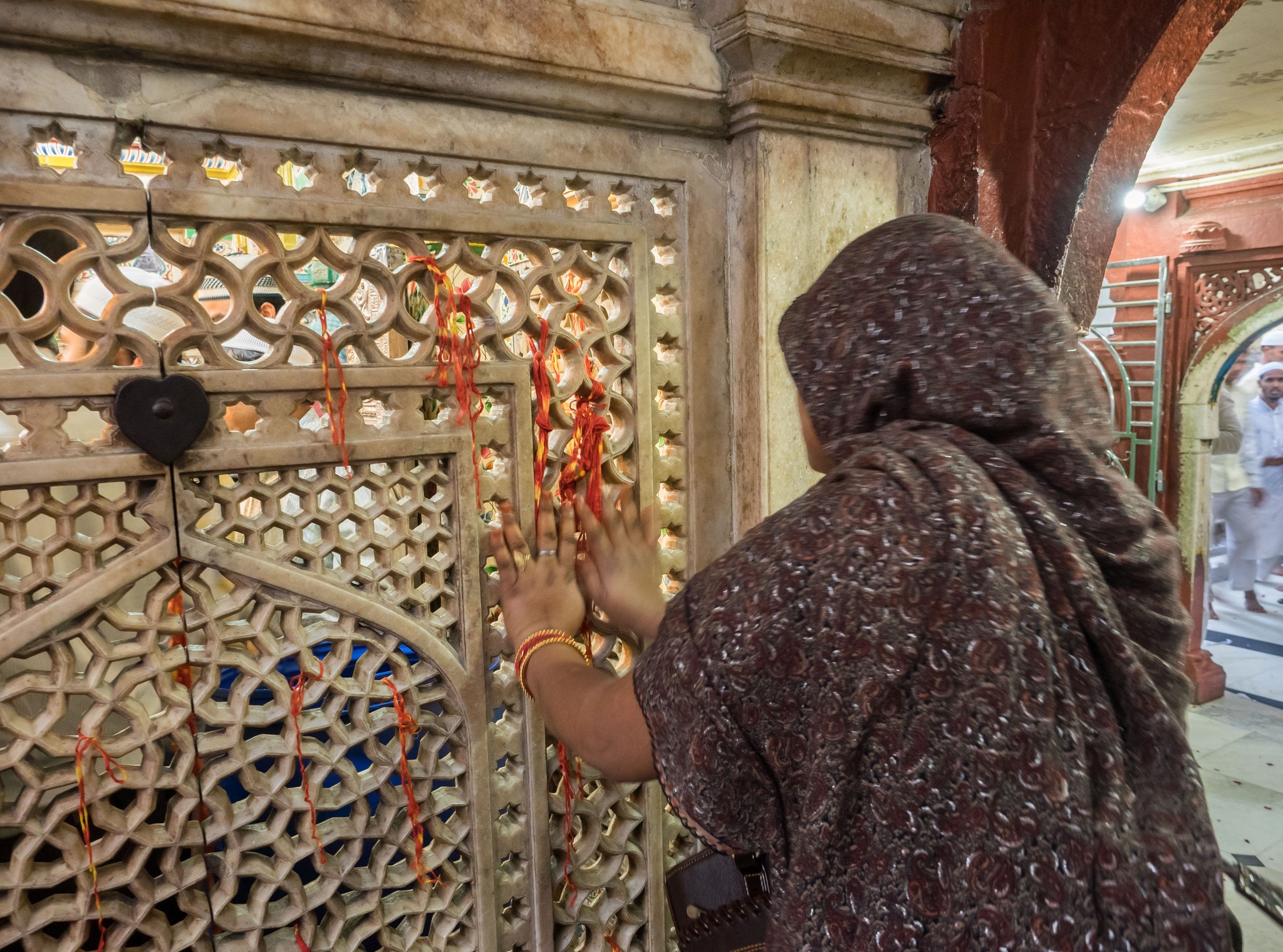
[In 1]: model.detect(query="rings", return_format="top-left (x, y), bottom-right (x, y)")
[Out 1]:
top-left (512, 550), bottom-right (531, 572)
top-left (536, 549), bottom-right (557, 555)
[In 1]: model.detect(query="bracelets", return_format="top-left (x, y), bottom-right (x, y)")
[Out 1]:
top-left (512, 629), bottom-right (591, 705)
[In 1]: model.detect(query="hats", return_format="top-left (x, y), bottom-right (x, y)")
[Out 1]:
top-left (1261, 332), bottom-right (1283, 346)
top-left (1259, 361), bottom-right (1283, 379)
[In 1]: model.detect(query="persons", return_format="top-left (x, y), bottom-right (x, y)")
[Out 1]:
top-left (491, 213), bottom-right (1244, 952)
top-left (1206, 332), bottom-right (1283, 620)
